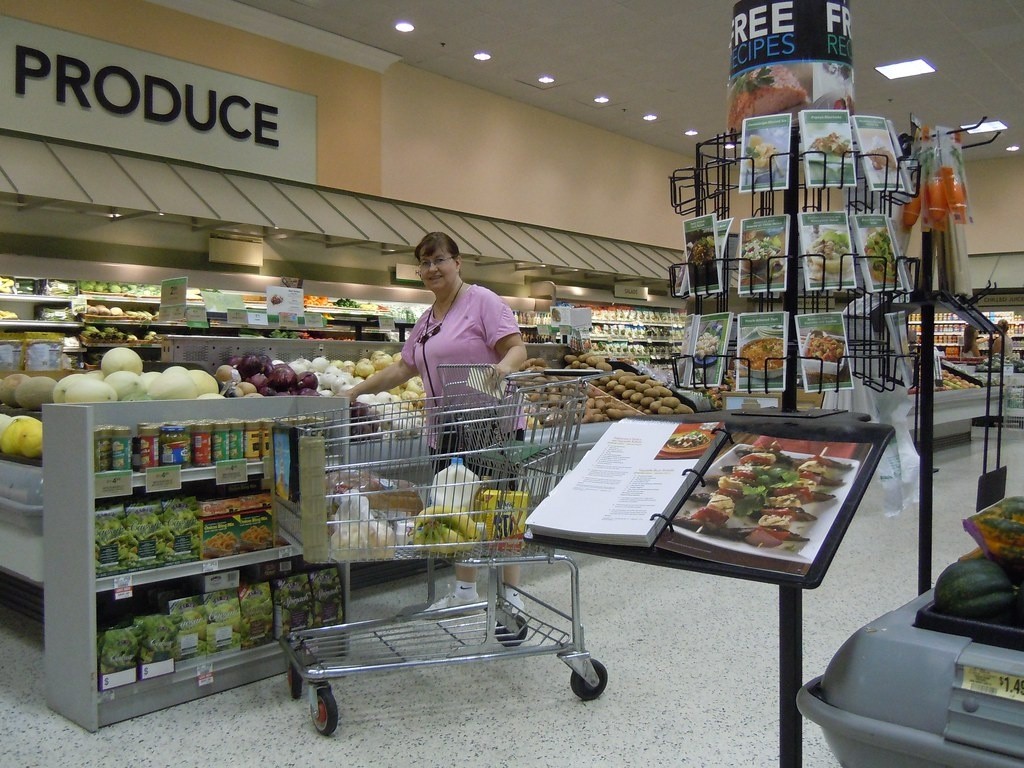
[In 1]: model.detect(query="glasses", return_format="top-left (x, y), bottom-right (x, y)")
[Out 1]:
top-left (418, 254), bottom-right (454, 269)
top-left (417, 323), bottom-right (442, 343)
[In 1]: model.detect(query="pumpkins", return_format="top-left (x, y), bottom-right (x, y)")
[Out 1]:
top-left (933, 495), bottom-right (1024, 628)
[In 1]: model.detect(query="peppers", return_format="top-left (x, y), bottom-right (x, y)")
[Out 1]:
top-left (690, 454), bottom-right (831, 540)
top-left (303, 295), bottom-right (360, 308)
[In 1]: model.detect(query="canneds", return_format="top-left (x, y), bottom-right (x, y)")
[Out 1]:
top-left (91, 416), bottom-right (330, 471)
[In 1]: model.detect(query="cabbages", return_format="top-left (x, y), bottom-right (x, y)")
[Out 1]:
top-left (78, 281), bottom-right (129, 293)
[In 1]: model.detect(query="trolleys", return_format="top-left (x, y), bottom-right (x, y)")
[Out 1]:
top-left (264, 366), bottom-right (615, 735)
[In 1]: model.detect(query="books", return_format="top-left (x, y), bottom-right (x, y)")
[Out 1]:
top-left (885, 311), bottom-right (915, 389)
top-left (738, 113), bottom-right (793, 194)
top-left (672, 213), bottom-right (734, 297)
top-left (795, 312), bottom-right (856, 393)
top-left (735, 312), bottom-right (790, 393)
top-left (797, 212), bottom-right (857, 292)
top-left (737, 215), bottom-right (791, 295)
top-left (678, 312), bottom-right (734, 387)
top-left (524, 416), bottom-right (874, 580)
top-left (850, 214), bottom-right (917, 293)
top-left (798, 109), bottom-right (857, 188)
top-left (850, 114), bottom-right (915, 205)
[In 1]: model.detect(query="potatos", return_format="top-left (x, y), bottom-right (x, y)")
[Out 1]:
top-left (516, 358), bottom-right (636, 427)
top-left (563, 352), bottom-right (695, 415)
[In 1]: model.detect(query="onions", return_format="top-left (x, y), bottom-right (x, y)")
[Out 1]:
top-left (228, 353), bottom-right (380, 442)
top-left (743, 529), bottom-right (783, 548)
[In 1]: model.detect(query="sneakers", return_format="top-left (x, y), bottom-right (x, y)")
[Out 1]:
top-left (503, 599), bottom-right (526, 620)
top-left (423, 585), bottom-right (480, 620)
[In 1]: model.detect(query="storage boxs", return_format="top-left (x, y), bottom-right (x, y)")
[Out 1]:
top-left (196, 499), bottom-right (241, 561)
top-left (483, 490), bottom-right (528, 552)
top-left (237, 489), bottom-right (274, 555)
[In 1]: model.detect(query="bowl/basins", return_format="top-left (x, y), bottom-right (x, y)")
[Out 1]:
top-left (739, 337), bottom-right (784, 379)
top-left (813, 255), bottom-right (850, 273)
top-left (802, 333), bottom-right (846, 375)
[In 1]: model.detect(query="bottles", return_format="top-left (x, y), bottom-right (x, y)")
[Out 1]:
top-left (430, 458), bottom-right (480, 520)
top-left (512, 310), bottom-right (551, 343)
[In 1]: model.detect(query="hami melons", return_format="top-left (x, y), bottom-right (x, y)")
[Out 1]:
top-left (0, 373), bottom-right (57, 410)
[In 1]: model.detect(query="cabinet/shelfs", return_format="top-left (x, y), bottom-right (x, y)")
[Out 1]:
top-left (39, 394), bottom-right (352, 735)
top-left (0, 291), bottom-right (567, 354)
top-left (590, 319), bottom-right (685, 361)
top-left (906, 320), bottom-right (1024, 351)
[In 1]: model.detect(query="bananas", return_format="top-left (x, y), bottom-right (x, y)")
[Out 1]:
top-left (406, 505), bottom-right (482, 553)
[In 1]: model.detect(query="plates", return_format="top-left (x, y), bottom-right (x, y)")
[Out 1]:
top-left (808, 137), bottom-right (853, 164)
top-left (661, 441), bottom-right (710, 452)
top-left (667, 444), bottom-right (860, 565)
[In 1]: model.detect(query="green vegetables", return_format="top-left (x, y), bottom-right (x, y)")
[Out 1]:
top-left (237, 329), bottom-right (299, 339)
top-left (866, 230), bottom-right (896, 278)
top-left (736, 66), bottom-right (775, 95)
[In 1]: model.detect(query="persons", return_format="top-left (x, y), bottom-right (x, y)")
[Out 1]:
top-left (992, 319), bottom-right (1014, 354)
top-left (334, 233), bottom-right (528, 621)
top-left (962, 326), bottom-right (989, 358)
